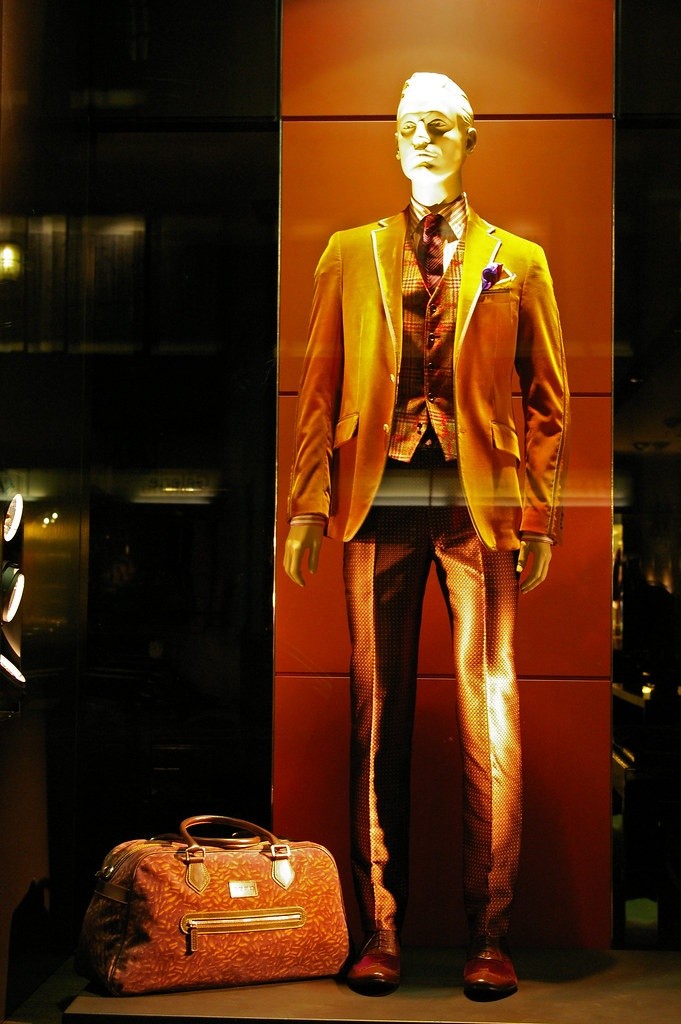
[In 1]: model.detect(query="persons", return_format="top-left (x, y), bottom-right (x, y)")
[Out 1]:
top-left (282, 69), bottom-right (571, 998)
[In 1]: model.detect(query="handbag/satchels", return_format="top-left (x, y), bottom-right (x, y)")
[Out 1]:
top-left (76, 814), bottom-right (350, 997)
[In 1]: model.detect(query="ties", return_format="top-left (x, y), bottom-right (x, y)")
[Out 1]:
top-left (417, 214), bottom-right (446, 293)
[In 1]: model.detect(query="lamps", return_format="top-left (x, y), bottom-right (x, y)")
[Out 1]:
top-left (0, 493), bottom-right (31, 714)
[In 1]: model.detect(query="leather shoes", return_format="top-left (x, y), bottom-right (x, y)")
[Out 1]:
top-left (463, 943), bottom-right (518, 994)
top-left (346, 930), bottom-right (400, 988)
